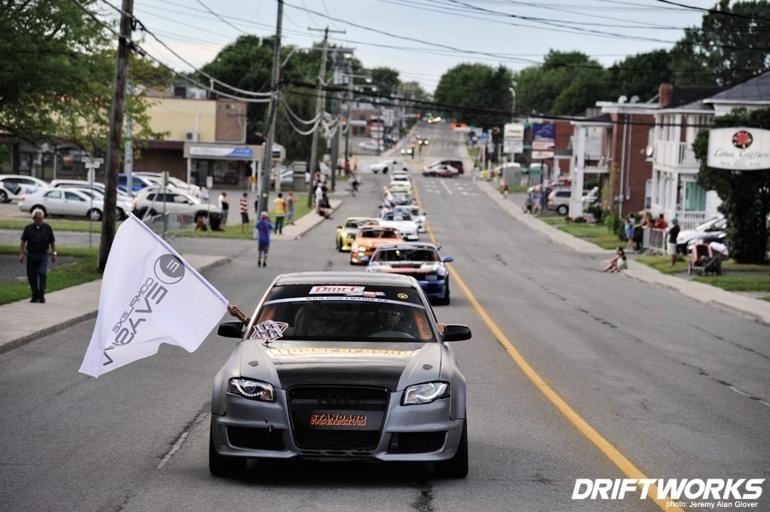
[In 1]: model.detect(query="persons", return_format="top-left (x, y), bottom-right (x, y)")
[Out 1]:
top-left (192, 144), bottom-right (362, 236)
top-left (255, 211), bottom-right (272, 269)
top-left (701, 237), bottom-right (730, 274)
top-left (490, 168), bottom-right (681, 275)
top-left (356, 303), bottom-right (416, 338)
top-left (16, 207), bottom-right (58, 304)
top-left (229, 302), bottom-right (337, 339)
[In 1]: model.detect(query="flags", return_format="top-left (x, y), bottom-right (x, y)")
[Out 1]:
top-left (77, 217), bottom-right (230, 374)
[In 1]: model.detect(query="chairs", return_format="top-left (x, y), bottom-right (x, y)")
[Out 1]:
top-left (687, 249), bottom-right (729, 276)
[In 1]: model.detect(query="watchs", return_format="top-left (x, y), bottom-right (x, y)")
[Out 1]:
top-left (51, 250), bottom-right (57, 256)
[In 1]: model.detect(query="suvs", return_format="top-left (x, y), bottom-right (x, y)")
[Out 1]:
top-left (547, 188), bottom-right (588, 215)
top-left (50, 180), bottom-right (129, 202)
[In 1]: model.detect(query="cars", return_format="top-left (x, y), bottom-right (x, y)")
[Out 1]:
top-left (400, 146), bottom-right (412, 155)
top-left (370, 157), bottom-right (465, 177)
top-left (337, 158), bottom-right (345, 168)
top-left (346, 225), bottom-right (407, 264)
top-left (359, 140), bottom-right (383, 152)
top-left (116, 171), bottom-right (224, 231)
top-left (380, 135), bottom-right (397, 145)
top-left (208, 270), bottom-right (475, 478)
top-left (582, 186), bottom-right (599, 206)
top-left (368, 242), bottom-right (455, 305)
top-left (455, 127), bottom-right (472, 133)
top-left (378, 171), bottom-right (427, 243)
top-left (0, 176), bottom-right (48, 203)
top-left (673, 216), bottom-right (725, 258)
top-left (528, 176), bottom-right (571, 195)
top-left (21, 188), bottom-right (103, 222)
top-left (77, 189), bottom-right (132, 221)
top-left (421, 113), bottom-right (441, 125)
top-left (334, 217), bottom-right (384, 253)
top-left (418, 136), bottom-right (430, 145)
top-left (683, 217), bottom-right (727, 256)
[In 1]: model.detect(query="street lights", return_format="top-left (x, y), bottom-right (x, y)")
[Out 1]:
top-left (330, 73), bottom-right (380, 170)
top-left (509, 87), bottom-right (515, 122)
top-left (309, 44), bottom-right (354, 180)
